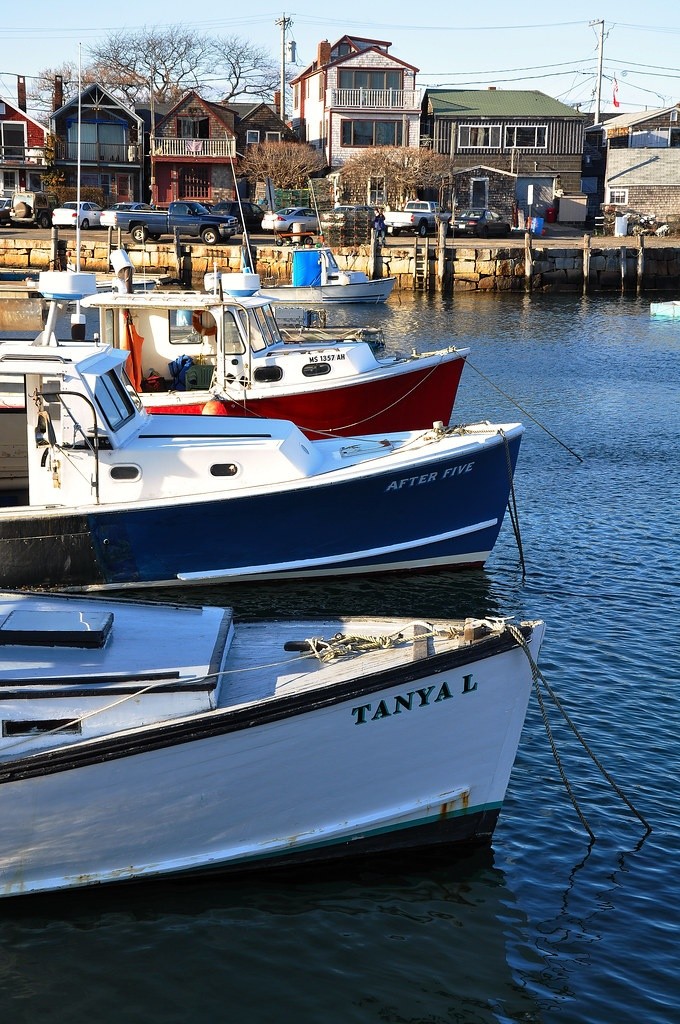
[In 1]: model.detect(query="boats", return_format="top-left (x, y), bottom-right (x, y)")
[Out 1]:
top-left (0, 233), bottom-right (473, 446)
top-left (1, 586), bottom-right (551, 906)
top-left (0, 270), bottom-right (530, 579)
top-left (193, 175), bottom-right (395, 309)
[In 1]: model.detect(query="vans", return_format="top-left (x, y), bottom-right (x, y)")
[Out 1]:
top-left (211, 201), bottom-right (273, 234)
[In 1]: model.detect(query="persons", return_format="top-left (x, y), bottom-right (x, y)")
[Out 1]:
top-left (372, 208), bottom-right (388, 248)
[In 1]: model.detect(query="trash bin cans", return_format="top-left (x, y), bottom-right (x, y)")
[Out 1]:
top-left (531, 216), bottom-right (544, 236)
top-left (546, 207), bottom-right (556, 223)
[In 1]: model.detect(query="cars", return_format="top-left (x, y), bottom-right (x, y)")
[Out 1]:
top-left (321, 205), bottom-right (377, 234)
top-left (0, 196), bottom-right (13, 225)
top-left (449, 207), bottom-right (511, 239)
top-left (51, 200), bottom-right (104, 230)
top-left (261, 206), bottom-right (320, 233)
top-left (100, 201), bottom-right (153, 230)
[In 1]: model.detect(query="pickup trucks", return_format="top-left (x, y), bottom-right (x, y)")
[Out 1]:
top-left (114, 201), bottom-right (241, 245)
top-left (382, 200), bottom-right (456, 238)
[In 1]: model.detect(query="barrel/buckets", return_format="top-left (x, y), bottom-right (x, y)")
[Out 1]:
top-left (350, 272), bottom-right (366, 282)
top-left (614, 217), bottom-right (627, 236)
top-left (110, 249), bottom-right (135, 282)
top-left (292, 249), bottom-right (322, 287)
top-left (531, 217), bottom-right (544, 236)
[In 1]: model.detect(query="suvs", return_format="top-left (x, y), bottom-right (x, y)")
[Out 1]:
top-left (11, 191), bottom-right (58, 227)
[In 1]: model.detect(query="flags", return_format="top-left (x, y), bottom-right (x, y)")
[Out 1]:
top-left (612, 78), bottom-right (619, 107)
